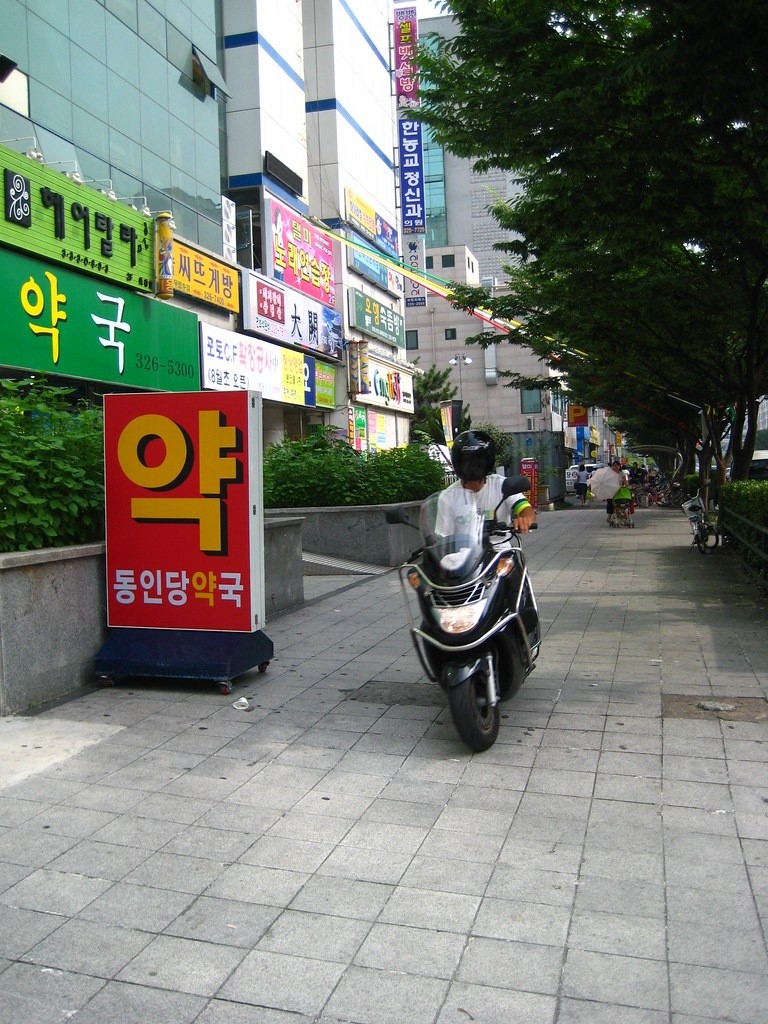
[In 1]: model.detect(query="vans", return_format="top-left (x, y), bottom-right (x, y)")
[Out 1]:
top-left (730, 450), bottom-right (768, 481)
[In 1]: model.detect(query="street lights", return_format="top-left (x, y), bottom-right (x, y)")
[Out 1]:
top-left (449, 352), bottom-right (472, 400)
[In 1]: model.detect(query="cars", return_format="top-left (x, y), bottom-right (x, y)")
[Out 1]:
top-left (569, 464), bottom-right (598, 475)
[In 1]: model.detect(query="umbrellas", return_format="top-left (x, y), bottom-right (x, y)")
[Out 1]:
top-left (590, 467), bottom-right (620, 500)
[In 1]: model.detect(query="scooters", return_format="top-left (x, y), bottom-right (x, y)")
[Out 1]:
top-left (385, 474), bottom-right (542, 754)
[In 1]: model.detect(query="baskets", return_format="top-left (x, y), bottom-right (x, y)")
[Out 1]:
top-left (681, 496), bottom-right (705, 518)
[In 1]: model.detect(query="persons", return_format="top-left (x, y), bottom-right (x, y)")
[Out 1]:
top-left (590, 468), bottom-right (597, 478)
top-left (606, 462), bottom-right (647, 523)
top-left (576, 464), bottom-right (589, 505)
top-left (433, 430), bottom-right (538, 634)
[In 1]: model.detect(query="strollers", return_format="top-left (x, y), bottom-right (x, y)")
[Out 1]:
top-left (609, 487), bottom-right (635, 529)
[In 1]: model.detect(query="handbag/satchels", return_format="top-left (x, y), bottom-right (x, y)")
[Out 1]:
top-left (573, 483), bottom-right (577, 489)
top-left (586, 490), bottom-right (593, 500)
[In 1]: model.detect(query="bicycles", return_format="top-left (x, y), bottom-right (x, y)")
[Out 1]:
top-left (633, 482), bottom-right (686, 508)
top-left (681, 486), bottom-right (719, 555)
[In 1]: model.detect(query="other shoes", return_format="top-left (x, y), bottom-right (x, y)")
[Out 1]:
top-left (520, 607), bottom-right (538, 635)
top-left (607, 519), bottom-right (611, 524)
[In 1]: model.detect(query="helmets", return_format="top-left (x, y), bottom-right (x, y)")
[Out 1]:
top-left (451, 430), bottom-right (497, 481)
